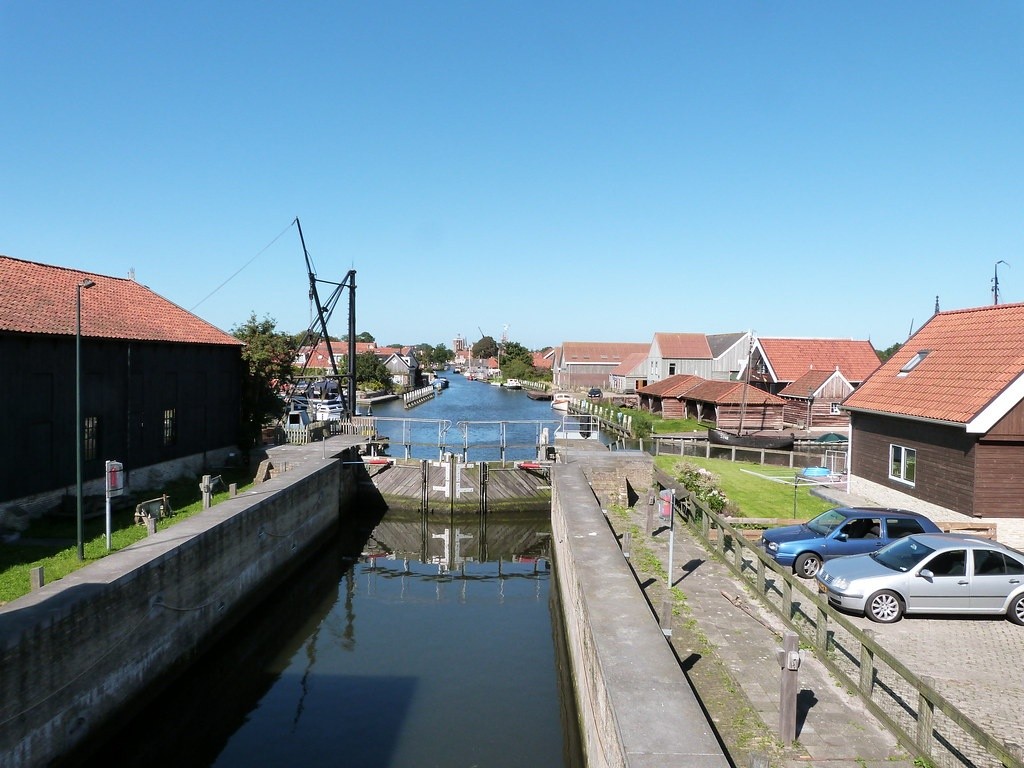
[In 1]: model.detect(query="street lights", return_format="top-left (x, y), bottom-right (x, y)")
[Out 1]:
top-left (74, 277), bottom-right (97, 562)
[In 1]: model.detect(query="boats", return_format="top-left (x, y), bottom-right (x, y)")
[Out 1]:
top-left (429, 374), bottom-right (449, 390)
top-left (268, 377), bottom-right (363, 437)
top-left (503, 378), bottom-right (524, 390)
top-left (549, 394), bottom-right (570, 410)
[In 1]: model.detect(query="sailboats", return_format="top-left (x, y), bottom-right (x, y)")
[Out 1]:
top-left (694, 333), bottom-right (795, 454)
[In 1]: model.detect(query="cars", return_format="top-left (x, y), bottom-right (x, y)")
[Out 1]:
top-left (759, 505), bottom-right (944, 580)
top-left (811, 533), bottom-right (1024, 628)
top-left (587, 388), bottom-right (604, 398)
